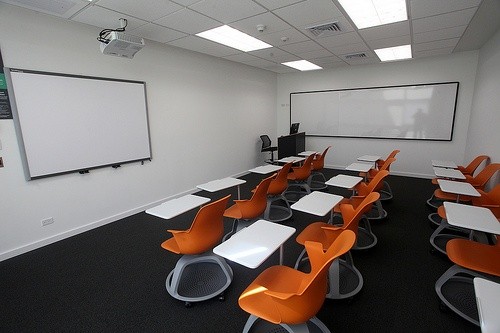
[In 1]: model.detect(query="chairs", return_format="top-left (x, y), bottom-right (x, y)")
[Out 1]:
top-left (426, 163), bottom-right (500, 208)
top-left (427, 184), bottom-right (500, 232)
top-left (328, 169), bottom-right (389, 250)
top-left (432, 156), bottom-right (489, 184)
top-left (359, 149), bottom-right (400, 178)
top-left (238, 230), bottom-right (357, 333)
top-left (251, 160), bottom-right (292, 222)
top-left (435, 236), bottom-right (500, 327)
top-left (429, 205), bottom-right (500, 255)
top-left (221, 173), bottom-right (278, 242)
top-left (160, 194), bottom-right (233, 302)
top-left (260, 135), bottom-right (278, 165)
top-left (294, 192), bottom-right (380, 300)
top-left (348, 158), bottom-right (398, 200)
top-left (301, 146), bottom-right (328, 190)
top-left (282, 153), bottom-right (316, 202)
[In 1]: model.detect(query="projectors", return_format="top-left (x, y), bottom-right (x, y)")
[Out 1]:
top-left (99, 31), bottom-right (144, 58)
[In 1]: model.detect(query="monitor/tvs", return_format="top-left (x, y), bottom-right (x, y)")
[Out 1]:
top-left (290, 123), bottom-right (299, 134)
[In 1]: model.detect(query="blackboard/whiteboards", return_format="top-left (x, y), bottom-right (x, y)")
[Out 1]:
top-left (3, 66), bottom-right (153, 182)
top-left (290, 81), bottom-right (459, 141)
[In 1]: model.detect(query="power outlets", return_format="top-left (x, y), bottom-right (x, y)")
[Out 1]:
top-left (41, 217), bottom-right (53, 226)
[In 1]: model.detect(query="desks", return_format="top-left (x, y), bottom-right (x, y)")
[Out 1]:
top-left (474, 276), bottom-right (500, 333)
top-left (325, 174), bottom-right (364, 196)
top-left (433, 167), bottom-right (466, 181)
top-left (298, 151), bottom-right (315, 156)
top-left (345, 162), bottom-right (373, 182)
top-left (278, 132), bottom-right (305, 173)
top-left (277, 156), bottom-right (306, 167)
top-left (432, 159), bottom-right (458, 169)
top-left (196, 177), bottom-right (247, 200)
top-left (437, 179), bottom-right (481, 203)
top-left (248, 164), bottom-right (284, 175)
top-left (290, 191), bottom-right (344, 225)
top-left (145, 194), bottom-right (211, 219)
top-left (357, 154), bottom-right (382, 169)
top-left (212, 218), bottom-right (296, 269)
top-left (442, 201), bottom-right (500, 240)
